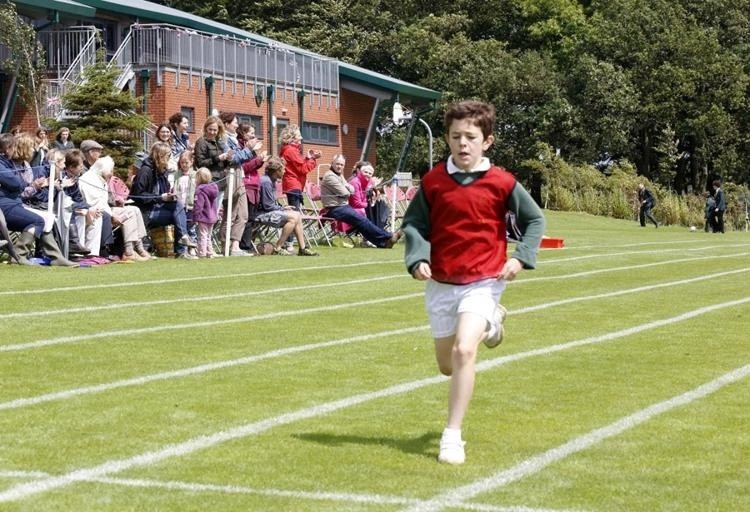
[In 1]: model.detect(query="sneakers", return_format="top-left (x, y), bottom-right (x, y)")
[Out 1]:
top-left (436, 425), bottom-right (467, 465)
top-left (119, 252), bottom-right (159, 264)
top-left (222, 249), bottom-right (254, 257)
top-left (298, 247), bottom-right (319, 256)
top-left (175, 254), bottom-right (199, 261)
top-left (483, 302), bottom-right (507, 349)
top-left (271, 249), bottom-right (292, 256)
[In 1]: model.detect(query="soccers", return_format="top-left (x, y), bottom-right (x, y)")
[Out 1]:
top-left (691, 226), bottom-right (696, 232)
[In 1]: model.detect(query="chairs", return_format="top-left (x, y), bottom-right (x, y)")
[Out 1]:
top-left (210, 222), bottom-right (224, 255)
top-left (246, 182), bottom-right (419, 256)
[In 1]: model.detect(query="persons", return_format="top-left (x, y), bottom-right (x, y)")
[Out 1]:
top-left (638, 182), bottom-right (659, 228)
top-left (704, 191), bottom-right (719, 232)
top-left (707, 179), bottom-right (725, 233)
top-left (399, 98), bottom-right (547, 465)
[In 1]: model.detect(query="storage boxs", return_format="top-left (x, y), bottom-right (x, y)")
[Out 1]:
top-left (541, 237), bottom-right (565, 248)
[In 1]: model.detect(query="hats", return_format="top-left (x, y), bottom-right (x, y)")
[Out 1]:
top-left (79, 139), bottom-right (103, 151)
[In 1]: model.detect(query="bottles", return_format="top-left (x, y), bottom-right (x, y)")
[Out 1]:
top-left (392, 173), bottom-right (399, 186)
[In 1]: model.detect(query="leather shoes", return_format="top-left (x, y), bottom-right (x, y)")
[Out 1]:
top-left (386, 228), bottom-right (402, 248)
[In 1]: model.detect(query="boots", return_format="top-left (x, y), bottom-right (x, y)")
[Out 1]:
top-left (10, 231), bottom-right (36, 265)
top-left (38, 231), bottom-right (78, 267)
